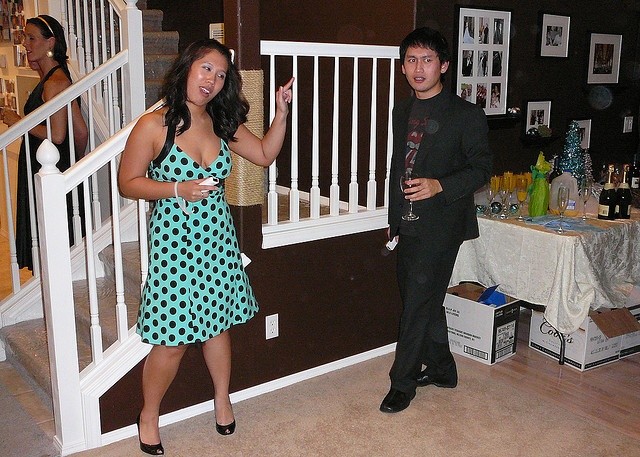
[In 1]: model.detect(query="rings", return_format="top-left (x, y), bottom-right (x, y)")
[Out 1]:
top-left (284, 90), bottom-right (288, 95)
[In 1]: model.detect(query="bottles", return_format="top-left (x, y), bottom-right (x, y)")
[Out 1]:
top-left (631, 152), bottom-right (639, 188)
top-left (617, 162), bottom-right (633, 219)
top-left (598, 163), bottom-right (616, 220)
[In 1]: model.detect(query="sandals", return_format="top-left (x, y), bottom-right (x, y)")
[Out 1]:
top-left (136, 413), bottom-right (165, 455)
top-left (214, 395), bottom-right (236, 435)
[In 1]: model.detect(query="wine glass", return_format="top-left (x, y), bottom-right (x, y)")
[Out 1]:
top-left (485, 180), bottom-right (496, 217)
top-left (556, 187), bottom-right (569, 234)
top-left (499, 177), bottom-right (510, 219)
top-left (399, 172), bottom-right (418, 221)
top-left (515, 180), bottom-right (528, 221)
top-left (579, 177), bottom-right (592, 220)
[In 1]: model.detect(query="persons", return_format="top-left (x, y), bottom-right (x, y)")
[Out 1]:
top-left (116, 37), bottom-right (295, 455)
top-left (0, 14), bottom-right (76, 276)
top-left (461, 15), bottom-right (502, 108)
top-left (377, 27), bottom-right (494, 413)
top-left (26, 53), bottom-right (89, 160)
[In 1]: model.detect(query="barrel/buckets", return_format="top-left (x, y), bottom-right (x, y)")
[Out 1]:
top-left (549, 169), bottom-right (580, 218)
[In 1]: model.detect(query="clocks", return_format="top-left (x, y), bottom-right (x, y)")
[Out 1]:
top-left (587, 86), bottom-right (613, 112)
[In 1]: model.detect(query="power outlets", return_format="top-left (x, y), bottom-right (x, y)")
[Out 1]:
top-left (265, 313), bottom-right (279, 340)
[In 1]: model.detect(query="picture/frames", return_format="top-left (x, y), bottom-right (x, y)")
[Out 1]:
top-left (451, 4), bottom-right (514, 121)
top-left (15, 75), bottom-right (40, 115)
top-left (586, 31), bottom-right (624, 86)
top-left (524, 98), bottom-right (553, 135)
top-left (536, 10), bottom-right (572, 61)
top-left (566, 118), bottom-right (592, 152)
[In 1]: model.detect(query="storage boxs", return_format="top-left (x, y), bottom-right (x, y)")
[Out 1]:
top-left (619, 308), bottom-right (640, 360)
top-left (443, 283), bottom-right (520, 366)
top-left (528, 307), bottom-right (640, 371)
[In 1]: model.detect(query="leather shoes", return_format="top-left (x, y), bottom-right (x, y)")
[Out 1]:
top-left (416, 367), bottom-right (457, 388)
top-left (380, 388), bottom-right (416, 413)
